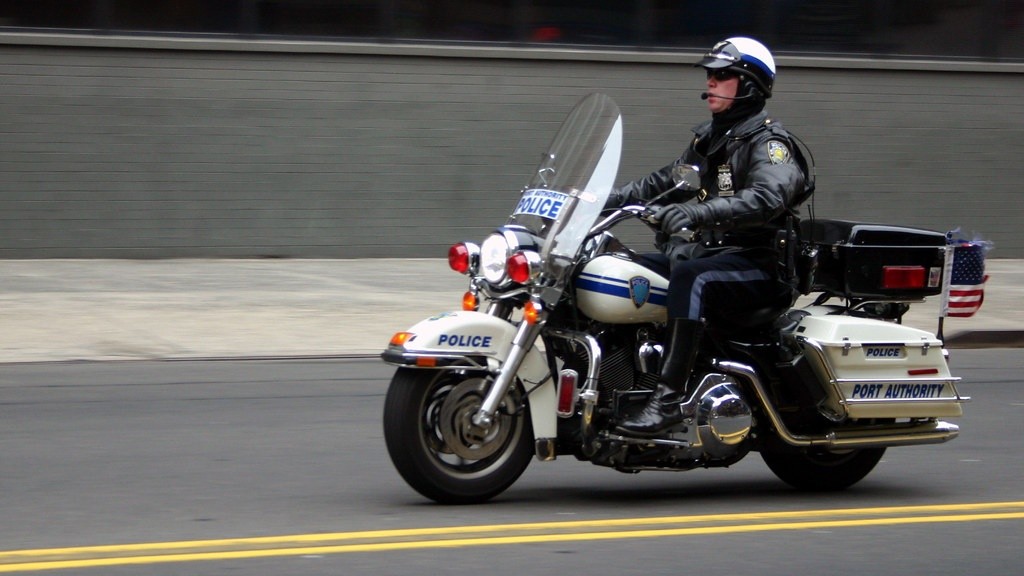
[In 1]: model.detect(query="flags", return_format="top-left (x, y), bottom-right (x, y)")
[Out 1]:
top-left (939, 246), bottom-right (984, 317)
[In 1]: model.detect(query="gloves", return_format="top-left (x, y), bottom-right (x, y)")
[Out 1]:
top-left (654, 196), bottom-right (732, 237)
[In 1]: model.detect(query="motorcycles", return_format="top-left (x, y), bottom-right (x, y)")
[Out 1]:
top-left (377, 89), bottom-right (994, 508)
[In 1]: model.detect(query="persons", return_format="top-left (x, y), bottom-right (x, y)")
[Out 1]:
top-left (592, 36), bottom-right (818, 437)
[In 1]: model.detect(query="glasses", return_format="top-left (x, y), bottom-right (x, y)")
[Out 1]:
top-left (706, 66), bottom-right (739, 82)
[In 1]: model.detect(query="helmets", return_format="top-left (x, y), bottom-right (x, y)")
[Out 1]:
top-left (694, 36), bottom-right (776, 98)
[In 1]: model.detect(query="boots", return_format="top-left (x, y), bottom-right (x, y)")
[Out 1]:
top-left (615, 317), bottom-right (706, 433)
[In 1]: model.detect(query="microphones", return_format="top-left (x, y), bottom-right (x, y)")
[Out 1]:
top-left (701, 92), bottom-right (751, 100)
